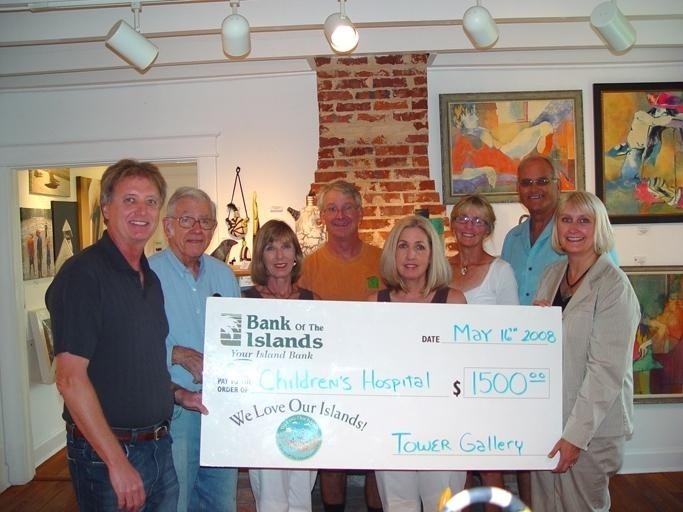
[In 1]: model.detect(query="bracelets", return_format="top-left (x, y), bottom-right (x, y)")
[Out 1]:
top-left (171, 387), bottom-right (184, 405)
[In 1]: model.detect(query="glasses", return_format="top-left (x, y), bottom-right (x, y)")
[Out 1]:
top-left (323, 206), bottom-right (357, 217)
top-left (519, 176), bottom-right (551, 188)
top-left (450, 215), bottom-right (489, 226)
top-left (169, 214), bottom-right (216, 231)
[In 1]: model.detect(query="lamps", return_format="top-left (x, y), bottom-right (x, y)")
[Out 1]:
top-left (590, 0), bottom-right (636, 52)
top-left (219, 1), bottom-right (251, 57)
top-left (324, 0), bottom-right (360, 54)
top-left (464, 0), bottom-right (498, 48)
top-left (104, 1), bottom-right (158, 72)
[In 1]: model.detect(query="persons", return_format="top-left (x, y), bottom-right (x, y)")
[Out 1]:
top-left (530, 190), bottom-right (642, 512)
top-left (447, 193), bottom-right (521, 512)
top-left (293, 180), bottom-right (389, 512)
top-left (500, 155), bottom-right (619, 507)
top-left (147, 186), bottom-right (242, 512)
top-left (45, 159), bottom-right (208, 512)
top-left (242, 219), bottom-right (322, 512)
top-left (366, 215), bottom-right (468, 512)
top-left (27, 231), bottom-right (51, 278)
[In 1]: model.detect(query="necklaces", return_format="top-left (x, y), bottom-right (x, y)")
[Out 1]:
top-left (565, 264), bottom-right (591, 288)
top-left (266, 284), bottom-right (295, 298)
top-left (460, 265), bottom-right (469, 276)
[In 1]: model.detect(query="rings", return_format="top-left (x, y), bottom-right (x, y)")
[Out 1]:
top-left (569, 466), bottom-right (573, 469)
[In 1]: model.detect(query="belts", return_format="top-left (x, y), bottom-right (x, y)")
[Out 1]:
top-left (64, 422), bottom-right (167, 443)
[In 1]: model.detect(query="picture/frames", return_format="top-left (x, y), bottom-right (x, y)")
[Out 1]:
top-left (439, 88), bottom-right (583, 206)
top-left (30, 307), bottom-right (58, 386)
top-left (616, 266), bottom-right (683, 403)
top-left (591, 83), bottom-right (683, 224)
